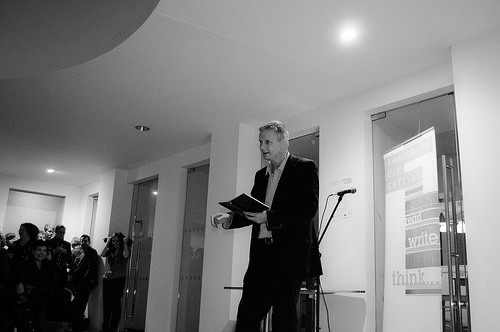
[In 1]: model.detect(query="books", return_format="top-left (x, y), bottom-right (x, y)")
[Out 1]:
top-left (218, 193), bottom-right (270, 217)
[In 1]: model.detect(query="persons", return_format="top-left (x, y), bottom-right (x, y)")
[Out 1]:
top-left (101, 232), bottom-right (133, 332)
top-left (211, 120), bottom-right (323, 332)
top-left (0, 223), bottom-right (99, 332)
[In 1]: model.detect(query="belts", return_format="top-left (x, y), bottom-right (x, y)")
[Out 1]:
top-left (257, 238), bottom-right (274, 245)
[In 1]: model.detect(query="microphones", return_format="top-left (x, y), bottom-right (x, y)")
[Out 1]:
top-left (337, 187), bottom-right (358, 196)
